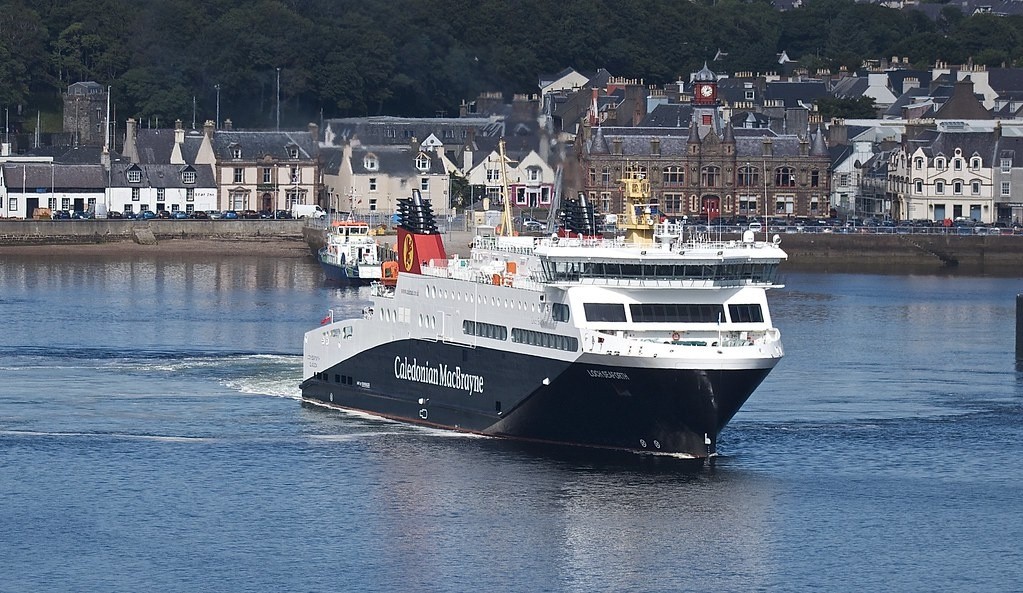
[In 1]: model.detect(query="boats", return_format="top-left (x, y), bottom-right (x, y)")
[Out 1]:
top-left (316, 181), bottom-right (393, 281)
top-left (299, 130), bottom-right (785, 473)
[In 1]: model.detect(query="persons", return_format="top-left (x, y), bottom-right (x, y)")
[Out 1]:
top-left (945, 218), bottom-right (953, 233)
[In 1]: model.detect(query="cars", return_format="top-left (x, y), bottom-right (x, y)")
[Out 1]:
top-left (240, 210), bottom-right (257, 219)
top-left (72, 210), bottom-right (91, 219)
top-left (224, 210), bottom-right (238, 218)
top-left (107, 211), bottom-right (120, 220)
top-left (686, 212), bottom-right (1023, 234)
top-left (53, 210), bottom-right (70, 219)
top-left (156, 210), bottom-right (170, 219)
top-left (170, 211), bottom-right (187, 220)
top-left (273, 211), bottom-right (290, 219)
top-left (136, 211), bottom-right (156, 219)
top-left (120, 210), bottom-right (137, 219)
top-left (205, 210), bottom-right (223, 221)
top-left (257, 209), bottom-right (273, 219)
top-left (190, 210), bottom-right (206, 219)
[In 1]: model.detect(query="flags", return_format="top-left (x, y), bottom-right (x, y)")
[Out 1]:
top-left (320, 313), bottom-right (330, 324)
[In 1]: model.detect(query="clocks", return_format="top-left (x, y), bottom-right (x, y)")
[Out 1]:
top-left (701, 85), bottom-right (713, 97)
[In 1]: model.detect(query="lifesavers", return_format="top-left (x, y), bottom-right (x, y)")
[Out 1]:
top-left (672, 332), bottom-right (681, 341)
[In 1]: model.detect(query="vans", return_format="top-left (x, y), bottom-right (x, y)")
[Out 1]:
top-left (292, 205), bottom-right (327, 221)
